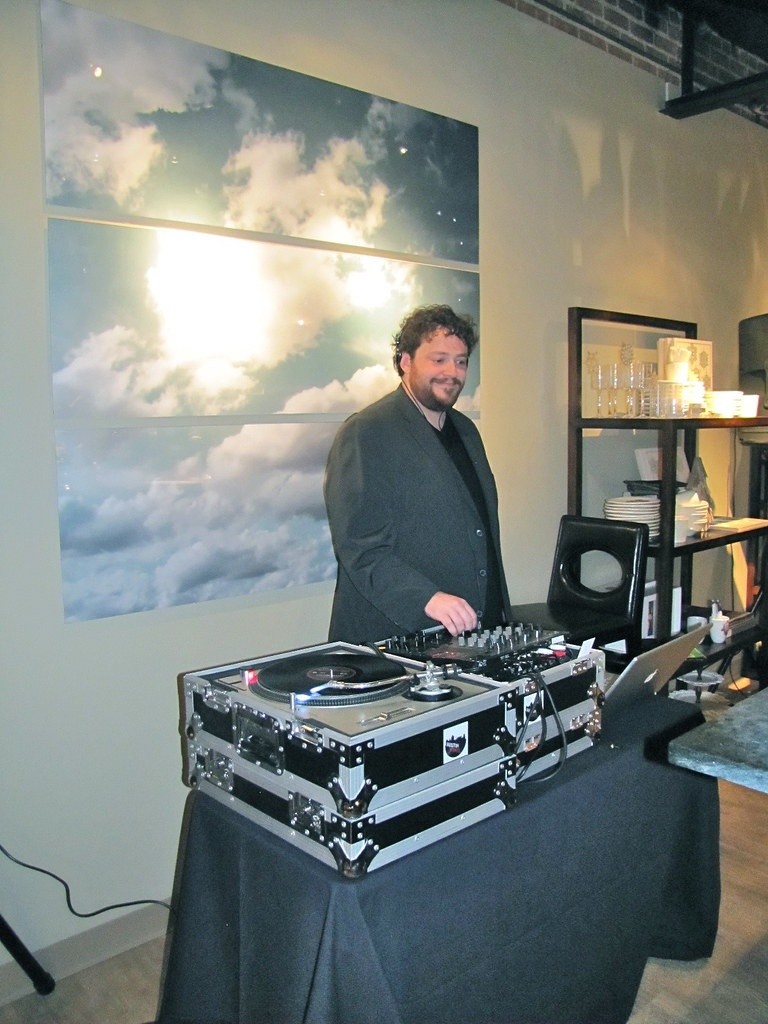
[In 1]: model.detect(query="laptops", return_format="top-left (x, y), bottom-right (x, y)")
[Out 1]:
top-left (603, 621), bottom-right (715, 706)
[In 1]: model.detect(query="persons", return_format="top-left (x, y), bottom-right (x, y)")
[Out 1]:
top-left (324, 304), bottom-right (514, 645)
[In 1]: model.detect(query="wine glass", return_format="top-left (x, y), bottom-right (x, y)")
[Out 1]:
top-left (593, 361), bottom-right (653, 417)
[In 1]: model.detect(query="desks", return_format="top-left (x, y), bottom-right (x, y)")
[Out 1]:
top-left (155, 694), bottom-right (721, 1024)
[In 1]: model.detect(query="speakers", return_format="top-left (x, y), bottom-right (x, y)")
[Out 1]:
top-left (739, 313), bottom-right (768, 447)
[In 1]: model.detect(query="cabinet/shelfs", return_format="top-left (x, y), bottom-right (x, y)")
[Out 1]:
top-left (567, 304), bottom-right (768, 699)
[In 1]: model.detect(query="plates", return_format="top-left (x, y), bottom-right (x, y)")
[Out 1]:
top-left (604, 497), bottom-right (661, 540)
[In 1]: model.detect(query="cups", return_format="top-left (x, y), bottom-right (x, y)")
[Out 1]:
top-left (675, 515), bottom-right (688, 544)
top-left (650, 380), bottom-right (704, 418)
top-left (740, 394), bottom-right (761, 417)
top-left (709, 615), bottom-right (729, 644)
top-left (687, 616), bottom-right (707, 644)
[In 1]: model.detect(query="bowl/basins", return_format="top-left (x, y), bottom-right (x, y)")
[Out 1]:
top-left (703, 390), bottom-right (743, 417)
top-left (674, 495), bottom-right (708, 536)
